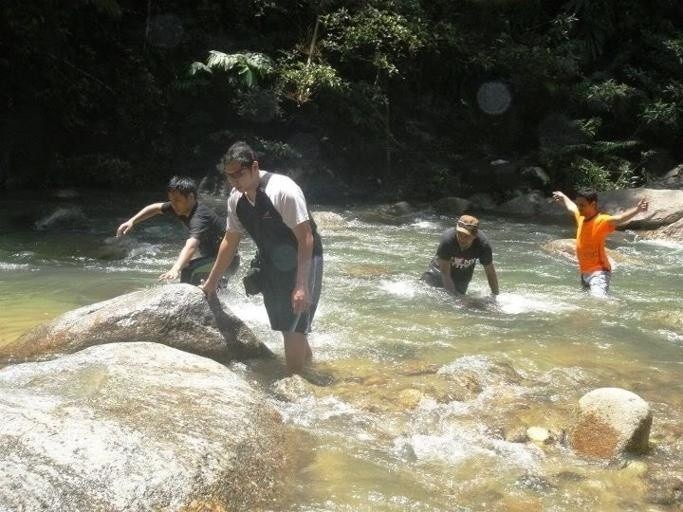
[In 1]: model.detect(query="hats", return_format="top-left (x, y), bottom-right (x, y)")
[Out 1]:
top-left (455, 214), bottom-right (480, 238)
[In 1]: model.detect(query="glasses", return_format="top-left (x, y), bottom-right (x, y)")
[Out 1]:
top-left (457, 220), bottom-right (477, 232)
top-left (226, 167), bottom-right (248, 179)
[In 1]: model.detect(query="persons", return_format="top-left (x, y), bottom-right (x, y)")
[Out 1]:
top-left (197, 142), bottom-right (325, 361)
top-left (549, 187), bottom-right (648, 296)
top-left (115, 174), bottom-right (240, 297)
top-left (418, 214), bottom-right (499, 298)
